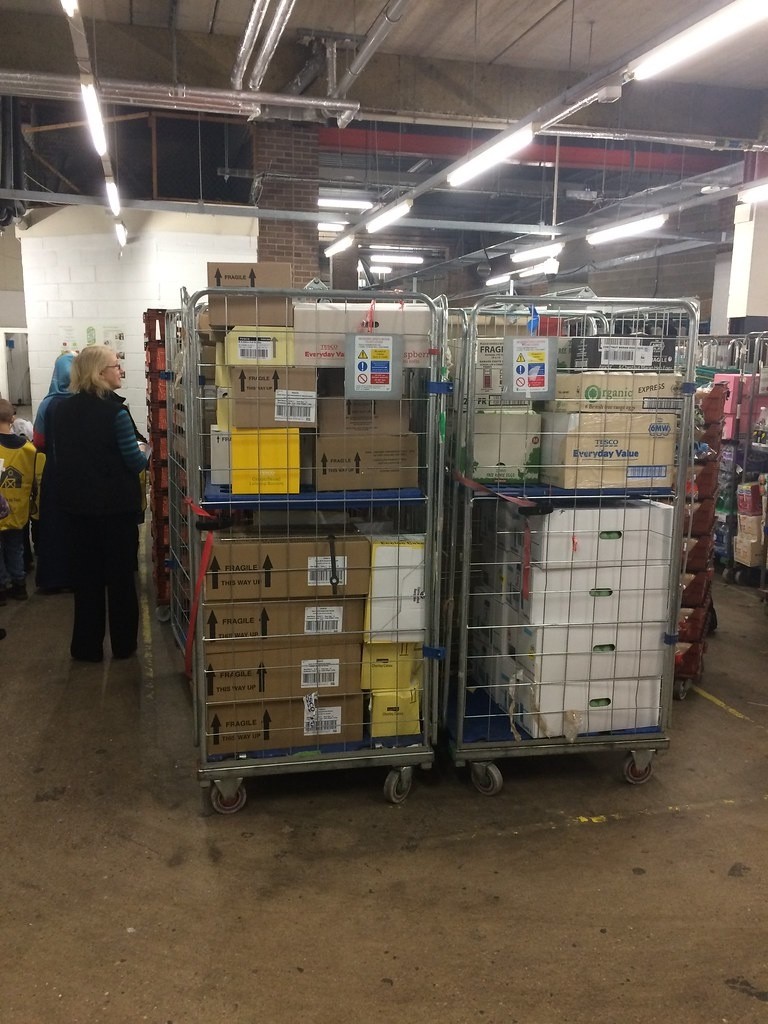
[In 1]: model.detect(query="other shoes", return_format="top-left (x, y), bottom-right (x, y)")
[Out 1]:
top-left (8, 584), bottom-right (28, 600)
top-left (0, 628), bottom-right (6, 640)
top-left (38, 587), bottom-right (59, 595)
top-left (0, 590), bottom-right (8, 606)
top-left (25, 562), bottom-right (35, 573)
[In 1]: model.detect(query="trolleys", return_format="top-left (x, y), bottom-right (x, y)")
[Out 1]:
top-left (443, 296), bottom-right (702, 799)
top-left (151, 294), bottom-right (452, 814)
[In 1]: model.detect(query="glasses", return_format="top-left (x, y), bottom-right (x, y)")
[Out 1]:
top-left (107, 363), bottom-right (121, 370)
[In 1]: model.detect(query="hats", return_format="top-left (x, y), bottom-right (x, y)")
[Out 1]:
top-left (13, 419), bottom-right (34, 441)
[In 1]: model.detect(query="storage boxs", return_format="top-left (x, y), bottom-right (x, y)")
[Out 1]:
top-left (148, 260), bottom-right (768, 761)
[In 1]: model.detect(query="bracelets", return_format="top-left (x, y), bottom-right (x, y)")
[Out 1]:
top-left (141, 450), bottom-right (146, 454)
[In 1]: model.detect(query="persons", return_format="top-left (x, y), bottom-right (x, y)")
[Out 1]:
top-left (0, 351), bottom-right (78, 607)
top-left (56, 346), bottom-right (152, 660)
